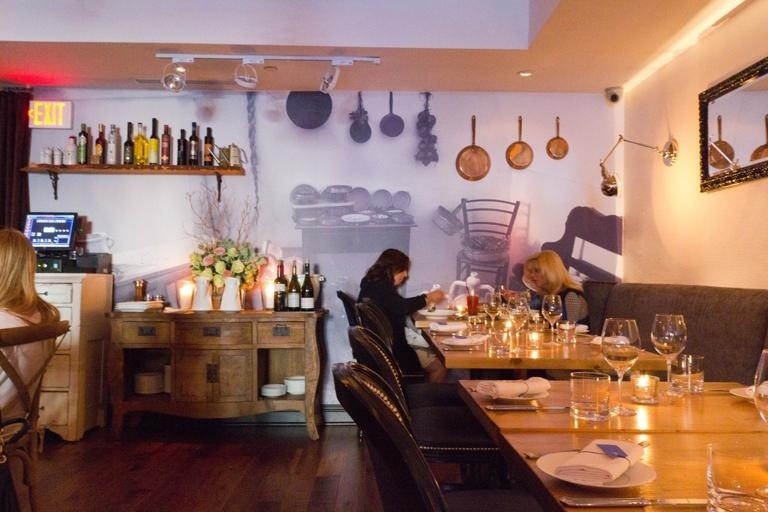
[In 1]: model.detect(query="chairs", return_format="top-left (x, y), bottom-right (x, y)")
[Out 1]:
top-left (356, 296), bottom-right (480, 404)
top-left (456, 197), bottom-right (518, 289)
top-left (347, 324), bottom-right (503, 485)
top-left (336, 289), bottom-right (357, 323)
top-left (0, 320), bottom-right (69, 512)
top-left (332, 359), bottom-right (546, 512)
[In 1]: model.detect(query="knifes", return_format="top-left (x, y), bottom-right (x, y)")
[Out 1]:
top-left (484, 404), bottom-right (569, 411)
top-left (559, 497), bottom-right (751, 506)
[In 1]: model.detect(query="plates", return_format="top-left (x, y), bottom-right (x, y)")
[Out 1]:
top-left (261, 376), bottom-right (306, 398)
top-left (418, 309), bottom-right (489, 349)
top-left (727, 386), bottom-right (754, 399)
top-left (291, 184), bottom-right (412, 226)
top-left (134, 365), bottom-right (171, 395)
top-left (499, 391), bottom-right (549, 400)
top-left (534, 450), bottom-right (657, 490)
top-left (115, 301), bottom-right (164, 312)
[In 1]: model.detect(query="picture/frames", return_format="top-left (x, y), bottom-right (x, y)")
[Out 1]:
top-left (697, 56), bottom-right (768, 193)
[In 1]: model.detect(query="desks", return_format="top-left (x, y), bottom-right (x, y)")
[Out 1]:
top-left (293, 209), bottom-right (416, 295)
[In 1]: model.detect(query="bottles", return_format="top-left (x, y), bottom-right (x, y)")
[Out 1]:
top-left (40, 119), bottom-right (214, 167)
top-left (274, 260), bottom-right (315, 310)
top-left (143, 294), bottom-right (162, 301)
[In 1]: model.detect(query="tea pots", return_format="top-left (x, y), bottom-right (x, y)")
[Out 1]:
top-left (208, 142), bottom-right (248, 168)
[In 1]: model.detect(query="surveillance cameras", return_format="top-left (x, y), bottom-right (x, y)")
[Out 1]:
top-left (607, 88), bottom-right (621, 103)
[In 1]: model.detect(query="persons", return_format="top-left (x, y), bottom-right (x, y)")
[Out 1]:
top-left (357, 248), bottom-right (450, 383)
top-left (525, 249), bottom-right (591, 335)
top-left (0, 226), bottom-right (60, 443)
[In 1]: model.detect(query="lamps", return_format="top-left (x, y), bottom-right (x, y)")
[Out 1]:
top-left (175, 280), bottom-right (194, 308)
top-left (260, 281), bottom-right (276, 310)
top-left (598, 133), bottom-right (679, 198)
top-left (154, 51), bottom-right (381, 95)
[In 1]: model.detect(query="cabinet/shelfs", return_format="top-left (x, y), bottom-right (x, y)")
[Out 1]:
top-left (29, 272), bottom-right (116, 455)
top-left (104, 308), bottom-right (326, 444)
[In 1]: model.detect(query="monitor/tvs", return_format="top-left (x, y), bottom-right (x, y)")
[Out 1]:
top-left (23, 212), bottom-right (78, 258)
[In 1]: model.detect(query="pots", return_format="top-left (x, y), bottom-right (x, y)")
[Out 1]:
top-left (456, 113), bottom-right (569, 182)
top-left (285, 90), bottom-right (403, 143)
top-left (710, 112), bottom-right (768, 169)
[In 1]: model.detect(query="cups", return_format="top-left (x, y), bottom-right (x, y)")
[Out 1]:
top-left (559, 320), bottom-right (576, 346)
top-left (670, 354), bottom-right (705, 396)
top-left (466, 279), bottom-right (481, 316)
top-left (706, 441), bottom-right (767, 511)
top-left (569, 371), bottom-right (611, 422)
top-left (753, 349), bottom-right (768, 425)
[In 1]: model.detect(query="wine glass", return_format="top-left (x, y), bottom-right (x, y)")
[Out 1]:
top-left (649, 313), bottom-right (688, 398)
top-left (483, 293), bottom-right (563, 353)
top-left (601, 317), bottom-right (642, 416)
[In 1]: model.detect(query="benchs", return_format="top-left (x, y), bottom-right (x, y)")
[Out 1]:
top-left (602, 284), bottom-right (768, 386)
top-left (539, 206), bottom-right (623, 282)
top-left (576, 279), bottom-right (613, 339)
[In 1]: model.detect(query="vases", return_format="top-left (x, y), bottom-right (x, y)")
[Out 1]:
top-left (213, 277), bottom-right (245, 311)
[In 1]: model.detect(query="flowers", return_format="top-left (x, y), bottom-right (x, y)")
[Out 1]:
top-left (190, 238), bottom-right (266, 291)
